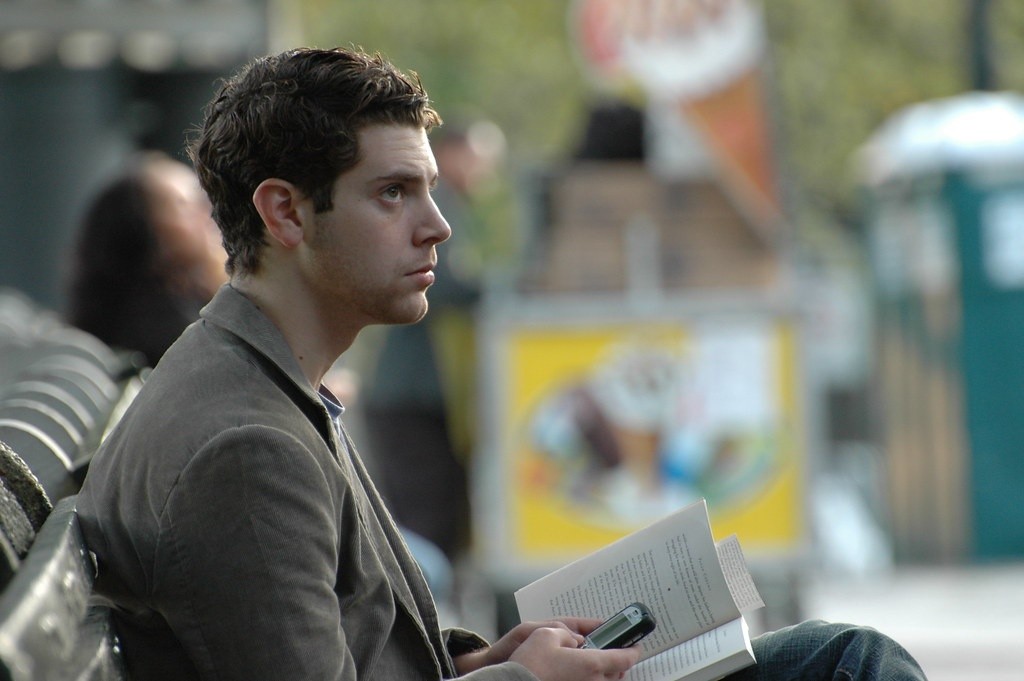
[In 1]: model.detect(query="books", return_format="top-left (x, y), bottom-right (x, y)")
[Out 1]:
top-left (514, 499), bottom-right (757, 681)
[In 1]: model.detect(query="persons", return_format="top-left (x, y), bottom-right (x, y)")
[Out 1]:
top-left (71, 126), bottom-right (489, 624)
top-left (73, 45), bottom-right (929, 681)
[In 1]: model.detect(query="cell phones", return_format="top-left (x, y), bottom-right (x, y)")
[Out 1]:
top-left (574, 602), bottom-right (659, 649)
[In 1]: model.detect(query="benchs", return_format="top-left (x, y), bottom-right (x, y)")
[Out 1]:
top-left (0, 290), bottom-right (146, 681)
